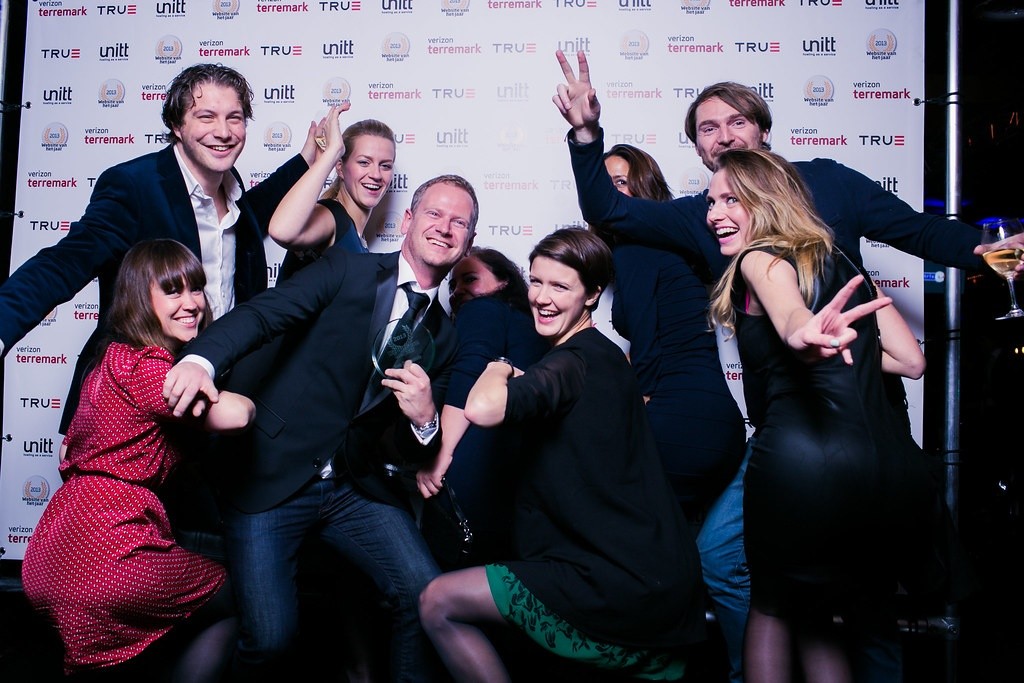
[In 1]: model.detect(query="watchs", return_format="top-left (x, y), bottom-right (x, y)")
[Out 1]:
top-left (492, 355), bottom-right (515, 379)
top-left (409, 414), bottom-right (438, 441)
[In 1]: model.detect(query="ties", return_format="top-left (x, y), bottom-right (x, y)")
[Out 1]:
top-left (357, 281), bottom-right (429, 419)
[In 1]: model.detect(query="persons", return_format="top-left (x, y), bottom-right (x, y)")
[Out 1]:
top-left (267, 101), bottom-right (398, 290)
top-left (1, 64), bottom-right (327, 547)
top-left (163, 172), bottom-right (479, 683)
top-left (705, 149), bottom-right (926, 683)
top-left (550, 48), bottom-right (1023, 682)
top-left (602, 143), bottom-right (747, 540)
top-left (420, 245), bottom-right (566, 683)
top-left (21, 237), bottom-right (256, 682)
top-left (417, 224), bottom-right (713, 683)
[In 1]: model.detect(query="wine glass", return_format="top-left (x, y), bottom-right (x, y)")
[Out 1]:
top-left (980, 217), bottom-right (1023, 321)
